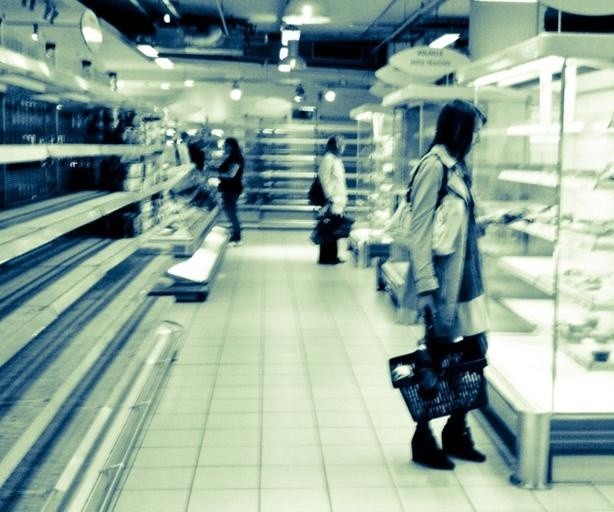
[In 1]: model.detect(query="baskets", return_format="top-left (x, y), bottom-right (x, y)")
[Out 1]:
top-left (388, 337), bottom-right (487, 422)
top-left (315, 214), bottom-right (354, 238)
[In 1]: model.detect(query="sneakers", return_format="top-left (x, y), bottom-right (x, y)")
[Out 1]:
top-left (226, 236), bottom-right (244, 247)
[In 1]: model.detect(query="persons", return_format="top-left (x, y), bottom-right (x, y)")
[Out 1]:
top-left (200, 138), bottom-right (244, 243)
top-left (180, 132), bottom-right (205, 173)
top-left (404, 98), bottom-right (525, 470)
top-left (316, 134), bottom-right (349, 264)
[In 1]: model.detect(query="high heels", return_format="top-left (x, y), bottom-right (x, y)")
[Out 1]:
top-left (441, 424), bottom-right (486, 463)
top-left (412, 427), bottom-right (455, 470)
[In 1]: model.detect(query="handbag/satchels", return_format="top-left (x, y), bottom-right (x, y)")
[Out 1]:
top-left (384, 194), bottom-right (466, 257)
top-left (307, 175), bottom-right (329, 206)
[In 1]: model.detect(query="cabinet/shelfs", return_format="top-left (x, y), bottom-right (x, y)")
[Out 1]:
top-left (374, 82), bottom-right (530, 323)
top-left (1, 27), bottom-right (183, 510)
top-left (173, 108), bottom-right (385, 230)
top-left (150, 118), bottom-right (230, 304)
top-left (448, 30), bottom-right (614, 491)
top-left (346, 102), bottom-right (408, 268)
